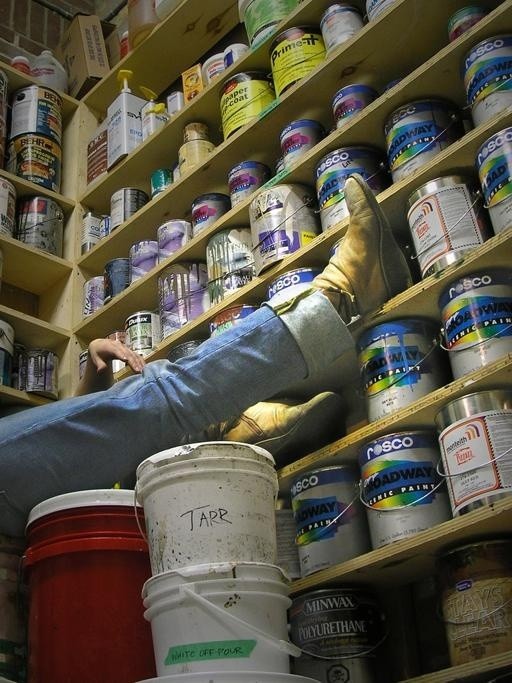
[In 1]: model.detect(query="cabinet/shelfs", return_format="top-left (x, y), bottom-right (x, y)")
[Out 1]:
top-left (3, 0), bottom-right (512, 681)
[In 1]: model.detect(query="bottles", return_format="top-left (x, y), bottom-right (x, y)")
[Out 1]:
top-left (12, 50), bottom-right (68, 92)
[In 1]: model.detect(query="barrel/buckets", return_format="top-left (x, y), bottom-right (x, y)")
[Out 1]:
top-left (436, 547), bottom-right (509, 674)
top-left (7, 2), bottom-right (510, 422)
top-left (133, 440), bottom-right (279, 562)
top-left (359, 432), bottom-right (445, 545)
top-left (438, 400), bottom-right (510, 517)
top-left (143, 565), bottom-right (293, 682)
top-left (294, 592), bottom-right (377, 679)
top-left (27, 488), bottom-right (152, 678)
top-left (289, 463), bottom-right (359, 568)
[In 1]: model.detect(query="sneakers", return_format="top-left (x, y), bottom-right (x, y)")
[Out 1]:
top-left (303, 172), bottom-right (413, 325)
top-left (206, 392), bottom-right (346, 458)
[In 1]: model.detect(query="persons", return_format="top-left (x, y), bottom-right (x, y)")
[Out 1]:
top-left (1, 168), bottom-right (412, 541)
